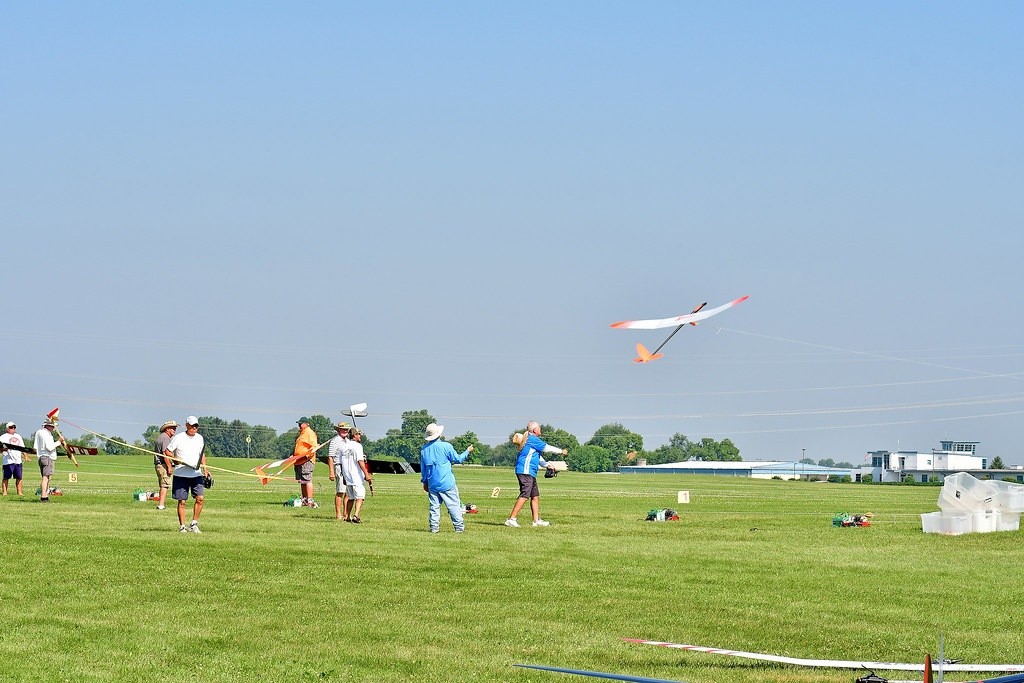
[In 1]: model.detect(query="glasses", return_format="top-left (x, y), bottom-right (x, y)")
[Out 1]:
top-left (9, 427), bottom-right (16, 429)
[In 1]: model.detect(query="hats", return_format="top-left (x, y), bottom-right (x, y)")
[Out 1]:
top-left (42, 419), bottom-right (58, 426)
top-left (6, 421), bottom-right (16, 428)
top-left (424, 423), bottom-right (444, 441)
top-left (348, 428), bottom-right (362, 435)
top-left (334, 422), bottom-right (351, 432)
top-left (160, 420), bottom-right (180, 432)
top-left (512, 431), bottom-right (529, 453)
top-left (186, 416), bottom-right (200, 426)
top-left (296, 417), bottom-right (309, 423)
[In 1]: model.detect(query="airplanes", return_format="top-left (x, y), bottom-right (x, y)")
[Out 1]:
top-left (611, 296), bottom-right (750, 364)
top-left (0, 407), bottom-right (99, 468)
top-left (58, 403), bottom-right (375, 497)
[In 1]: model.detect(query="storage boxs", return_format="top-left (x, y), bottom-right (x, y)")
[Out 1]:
top-left (920, 471), bottom-right (1024, 536)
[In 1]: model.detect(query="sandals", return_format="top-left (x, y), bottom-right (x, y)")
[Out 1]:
top-left (352, 515), bottom-right (361, 523)
top-left (344, 517), bottom-right (351, 522)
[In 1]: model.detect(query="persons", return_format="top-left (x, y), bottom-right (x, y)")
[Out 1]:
top-left (328, 422), bottom-right (371, 523)
top-left (154, 421), bottom-right (180, 509)
top-left (0, 422), bottom-right (29, 496)
top-left (34, 419), bottom-right (64, 502)
top-left (165, 415), bottom-right (211, 532)
top-left (421, 424), bottom-right (474, 532)
top-left (505, 422), bottom-right (567, 527)
top-left (289, 417), bottom-right (317, 506)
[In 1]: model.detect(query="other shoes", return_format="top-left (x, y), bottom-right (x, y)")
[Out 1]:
top-left (189, 523), bottom-right (202, 533)
top-left (505, 520), bottom-right (522, 528)
top-left (41, 497), bottom-right (50, 502)
top-left (179, 527), bottom-right (188, 532)
top-left (532, 521), bottom-right (549, 527)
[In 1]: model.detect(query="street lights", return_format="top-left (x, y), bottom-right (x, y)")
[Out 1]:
top-left (932, 448), bottom-right (935, 482)
top-left (802, 448), bottom-right (805, 472)
top-left (794, 462), bottom-right (797, 478)
